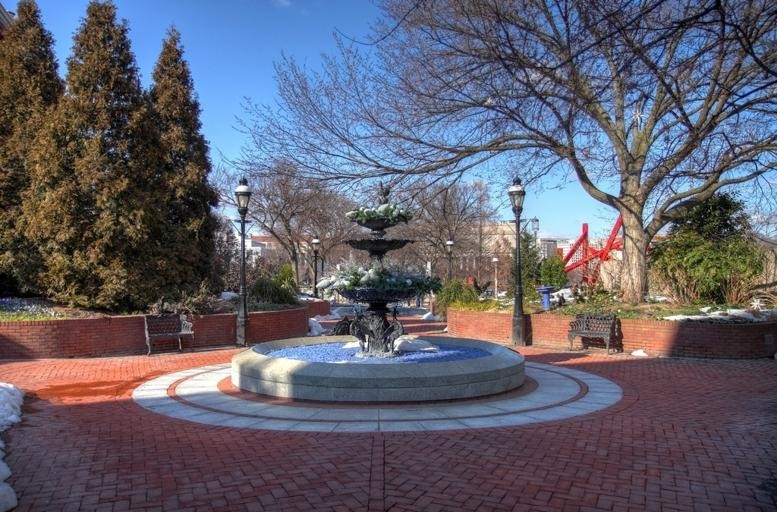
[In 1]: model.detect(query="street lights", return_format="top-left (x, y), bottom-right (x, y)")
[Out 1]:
top-left (507, 174), bottom-right (532, 346)
top-left (446, 238), bottom-right (454, 280)
top-left (311, 233), bottom-right (321, 298)
top-left (491, 253), bottom-right (500, 298)
top-left (235, 174), bottom-right (253, 347)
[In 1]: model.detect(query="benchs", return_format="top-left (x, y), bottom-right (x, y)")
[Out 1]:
top-left (568, 311), bottom-right (617, 353)
top-left (145, 313), bottom-right (195, 356)
